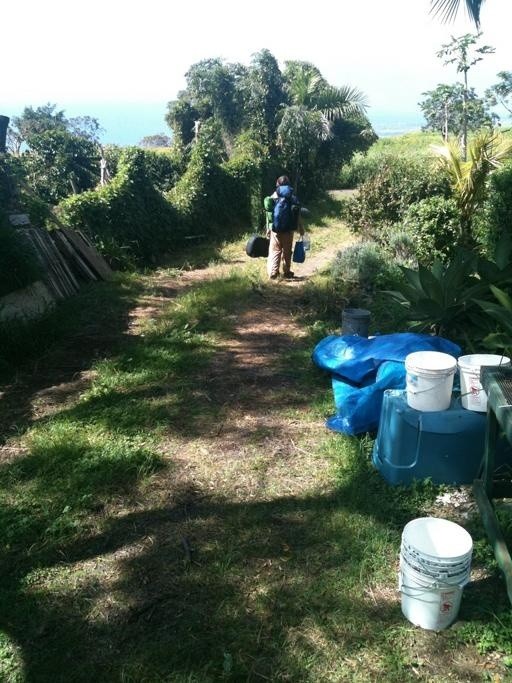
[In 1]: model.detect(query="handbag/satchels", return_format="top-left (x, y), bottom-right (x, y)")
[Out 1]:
top-left (246, 237), bottom-right (269, 257)
top-left (293, 242), bottom-right (305, 262)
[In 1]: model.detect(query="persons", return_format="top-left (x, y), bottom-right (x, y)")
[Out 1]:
top-left (265, 175), bottom-right (304, 280)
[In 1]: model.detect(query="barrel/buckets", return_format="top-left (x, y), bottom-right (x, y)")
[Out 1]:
top-left (398, 517), bottom-right (473, 630)
top-left (405, 351), bottom-right (457, 412)
top-left (458, 354), bottom-right (512, 413)
top-left (341, 308), bottom-right (371, 339)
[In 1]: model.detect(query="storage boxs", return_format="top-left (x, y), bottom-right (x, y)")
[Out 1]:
top-left (372, 388), bottom-right (512, 488)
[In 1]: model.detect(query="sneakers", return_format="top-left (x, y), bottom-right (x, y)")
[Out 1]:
top-left (271, 273), bottom-right (277, 279)
top-left (281, 272), bottom-right (294, 278)
